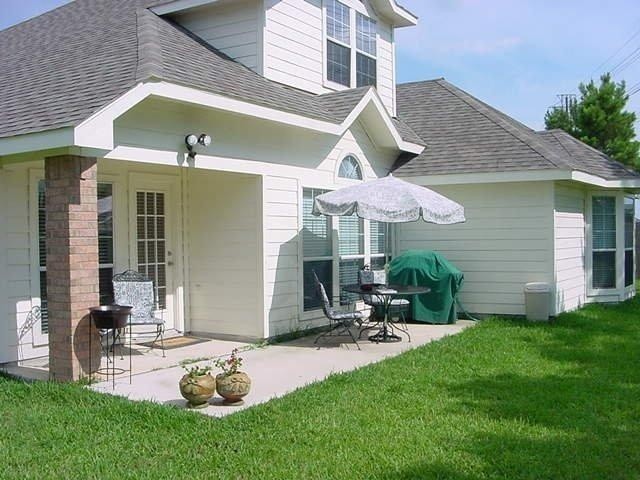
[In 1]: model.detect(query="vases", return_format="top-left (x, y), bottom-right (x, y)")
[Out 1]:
top-left (217, 372), bottom-right (251, 406)
top-left (178, 375), bottom-right (216, 410)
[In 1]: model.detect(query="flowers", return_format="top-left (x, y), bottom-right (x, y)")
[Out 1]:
top-left (181, 364), bottom-right (213, 377)
top-left (213, 348), bottom-right (243, 376)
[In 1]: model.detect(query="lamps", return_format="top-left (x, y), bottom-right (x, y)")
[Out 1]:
top-left (183, 133), bottom-right (212, 158)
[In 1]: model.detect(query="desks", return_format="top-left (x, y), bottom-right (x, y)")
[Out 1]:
top-left (342, 283), bottom-right (432, 343)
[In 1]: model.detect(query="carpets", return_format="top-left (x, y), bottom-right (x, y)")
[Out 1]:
top-left (136, 335), bottom-right (212, 350)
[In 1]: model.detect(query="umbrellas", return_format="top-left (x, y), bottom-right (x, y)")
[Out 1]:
top-left (310, 173), bottom-right (466, 288)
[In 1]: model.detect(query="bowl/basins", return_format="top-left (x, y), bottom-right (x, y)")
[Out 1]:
top-left (89, 305), bottom-right (133, 329)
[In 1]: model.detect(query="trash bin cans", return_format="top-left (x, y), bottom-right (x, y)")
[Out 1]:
top-left (525, 282), bottom-right (550, 323)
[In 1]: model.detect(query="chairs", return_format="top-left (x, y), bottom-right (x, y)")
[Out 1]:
top-left (109, 268), bottom-right (165, 361)
top-left (311, 268), bottom-right (364, 350)
top-left (358, 269), bottom-right (410, 331)
